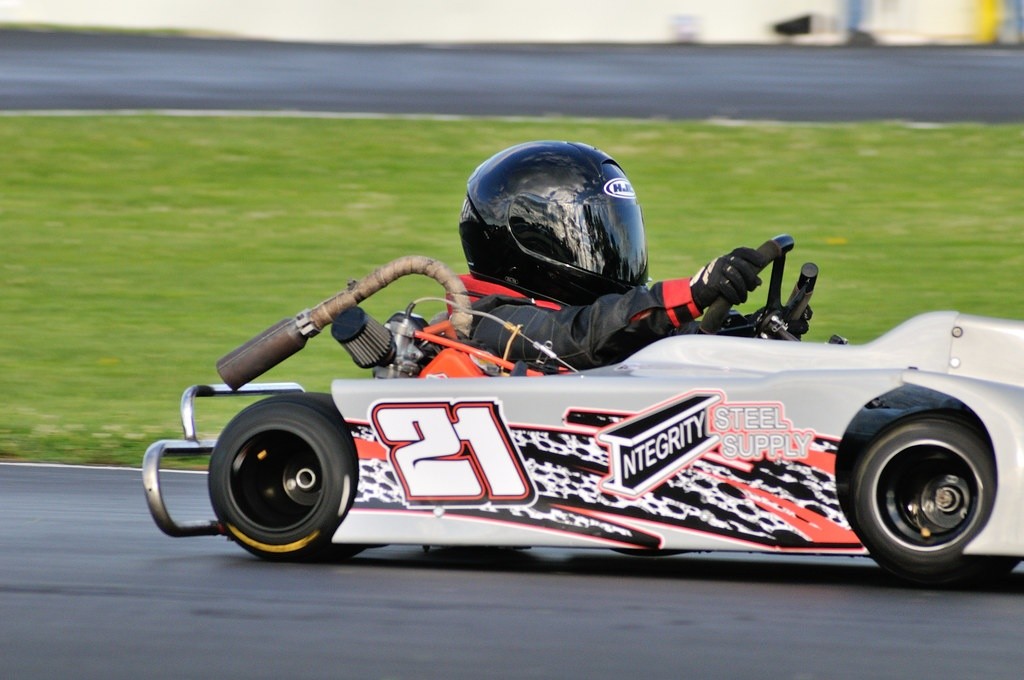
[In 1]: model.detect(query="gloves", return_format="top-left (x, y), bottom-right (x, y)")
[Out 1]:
top-left (689, 246), bottom-right (765, 312)
top-left (743, 301), bottom-right (813, 343)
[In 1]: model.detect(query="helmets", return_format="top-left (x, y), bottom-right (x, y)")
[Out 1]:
top-left (459, 139), bottom-right (648, 306)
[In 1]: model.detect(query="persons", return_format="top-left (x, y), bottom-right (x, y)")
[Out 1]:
top-left (457, 141), bottom-right (813, 376)
